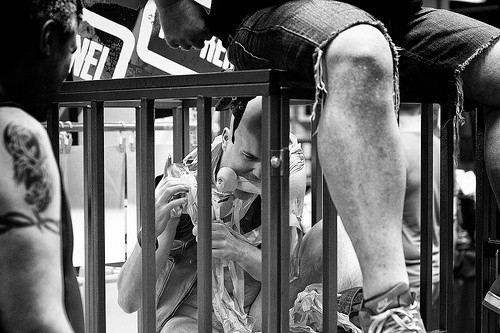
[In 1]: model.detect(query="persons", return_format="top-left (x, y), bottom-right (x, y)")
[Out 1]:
top-left (154, 0), bottom-right (500, 333)
top-left (384, 101), bottom-right (461, 264)
top-left (0, 0), bottom-right (106, 333)
top-left (116, 92), bottom-right (364, 332)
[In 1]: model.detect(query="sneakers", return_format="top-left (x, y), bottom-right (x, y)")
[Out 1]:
top-left (359, 280), bottom-right (427, 333)
top-left (481, 275), bottom-right (500, 315)
